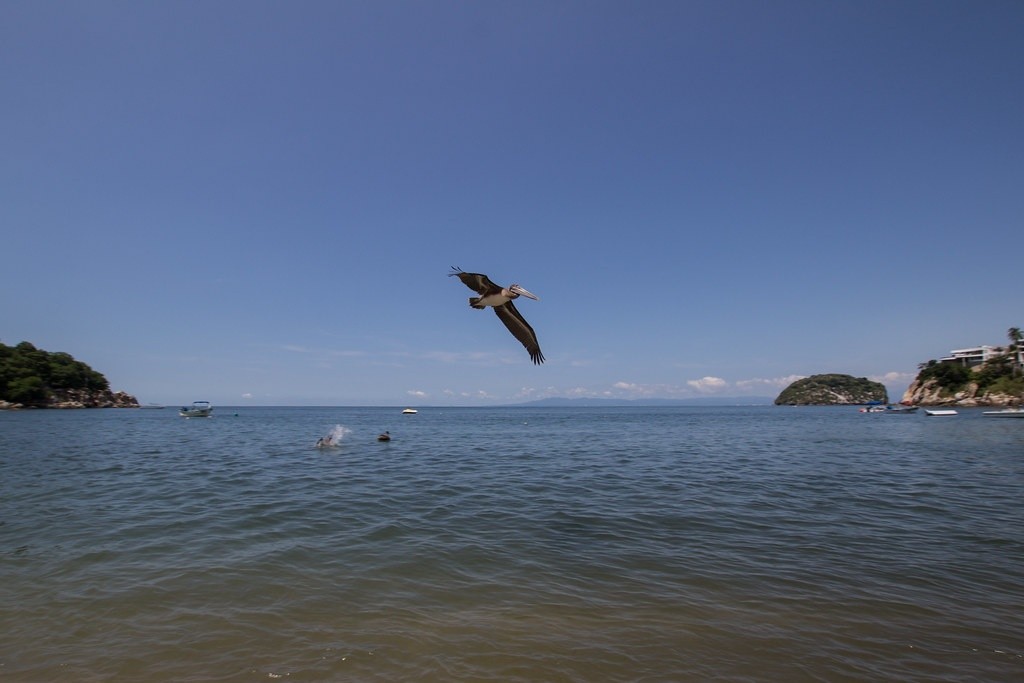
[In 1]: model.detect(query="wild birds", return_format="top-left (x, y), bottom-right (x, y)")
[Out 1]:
top-left (448, 264), bottom-right (546, 365)
top-left (377, 431), bottom-right (391, 442)
top-left (315, 434), bottom-right (334, 445)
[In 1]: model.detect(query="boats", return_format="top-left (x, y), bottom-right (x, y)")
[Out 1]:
top-left (925, 409), bottom-right (959, 417)
top-left (981, 409), bottom-right (1024, 418)
top-left (860, 404), bottom-right (921, 415)
top-left (402, 408), bottom-right (419, 415)
top-left (178, 401), bottom-right (213, 419)
